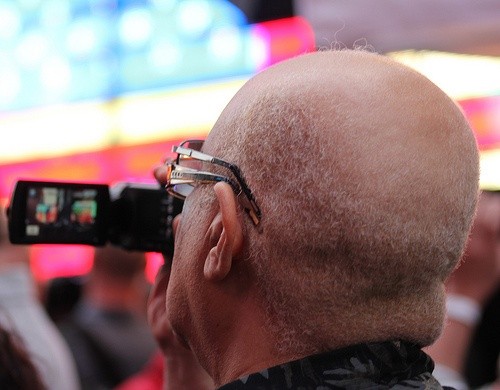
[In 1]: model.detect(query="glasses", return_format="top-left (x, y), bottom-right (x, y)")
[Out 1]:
top-left (166, 157), bottom-right (261, 227)
top-left (171, 138), bottom-right (261, 218)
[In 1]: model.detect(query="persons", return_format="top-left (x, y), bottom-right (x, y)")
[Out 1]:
top-left (148, 50), bottom-right (479, 390)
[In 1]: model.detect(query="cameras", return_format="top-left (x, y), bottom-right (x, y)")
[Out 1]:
top-left (8, 180), bottom-right (185, 256)
